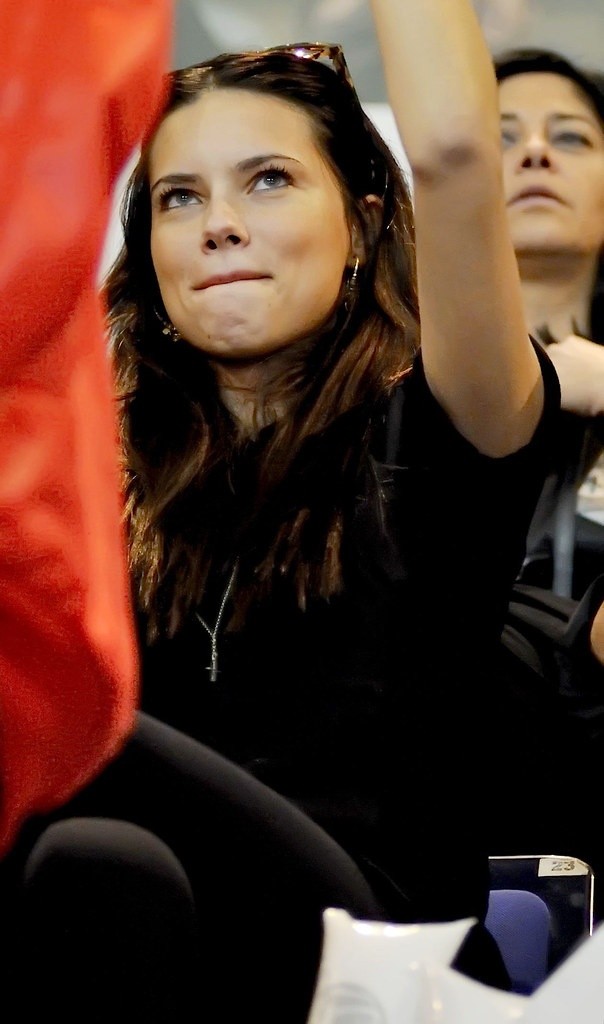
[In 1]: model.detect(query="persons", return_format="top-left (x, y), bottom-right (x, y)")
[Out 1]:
top-left (0, 1), bottom-right (561, 1024)
top-left (492, 49), bottom-right (604, 928)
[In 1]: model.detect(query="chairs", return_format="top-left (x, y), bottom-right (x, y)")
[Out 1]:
top-left (483, 853), bottom-right (595, 999)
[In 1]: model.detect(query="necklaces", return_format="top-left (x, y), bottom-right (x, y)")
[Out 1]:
top-left (195, 568), bottom-right (238, 682)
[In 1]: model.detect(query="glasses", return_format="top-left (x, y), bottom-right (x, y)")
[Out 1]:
top-left (258, 40), bottom-right (358, 105)
